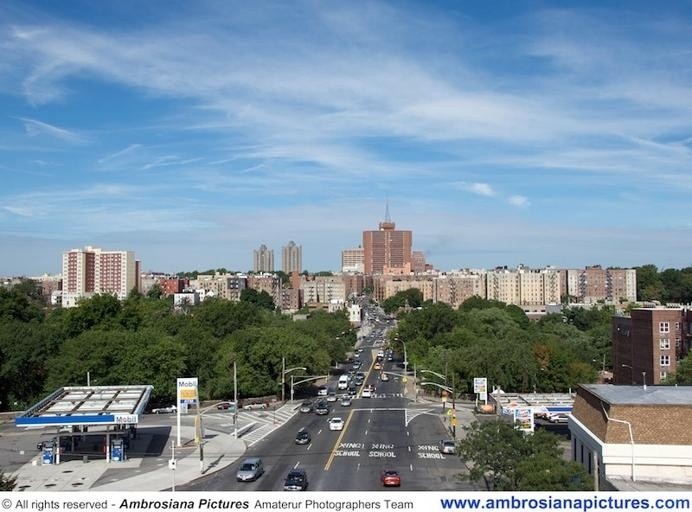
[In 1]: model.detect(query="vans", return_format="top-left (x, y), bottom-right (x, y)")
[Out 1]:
top-left (337, 374), bottom-right (349, 390)
top-left (236, 457), bottom-right (264, 482)
top-left (317, 383), bottom-right (332, 396)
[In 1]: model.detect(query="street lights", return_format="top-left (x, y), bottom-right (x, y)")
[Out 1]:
top-left (420, 368), bottom-right (446, 412)
top-left (419, 382), bottom-right (456, 440)
top-left (198, 401), bottom-right (237, 472)
top-left (281, 356), bottom-right (307, 401)
top-left (620, 364), bottom-right (633, 384)
top-left (591, 358), bottom-right (605, 375)
top-left (393, 337), bottom-right (408, 395)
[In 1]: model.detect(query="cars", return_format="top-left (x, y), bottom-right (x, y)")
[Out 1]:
top-left (326, 391), bottom-right (337, 401)
top-left (548, 412), bottom-right (569, 423)
top-left (151, 404), bottom-right (177, 415)
top-left (36, 434), bottom-right (82, 450)
top-left (437, 439), bottom-right (457, 454)
top-left (349, 295), bottom-right (392, 385)
top-left (379, 468), bottom-right (401, 487)
top-left (300, 398), bottom-right (314, 413)
top-left (326, 417), bottom-right (345, 431)
top-left (294, 430), bottom-right (311, 446)
top-left (340, 396), bottom-right (352, 407)
top-left (348, 385), bottom-right (357, 395)
top-left (364, 382), bottom-right (376, 393)
top-left (316, 400), bottom-right (331, 415)
top-left (217, 401), bottom-right (243, 409)
top-left (244, 401), bottom-right (270, 410)
top-left (361, 388), bottom-right (371, 398)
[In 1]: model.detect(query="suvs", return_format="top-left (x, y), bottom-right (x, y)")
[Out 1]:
top-left (283, 467), bottom-right (308, 491)
